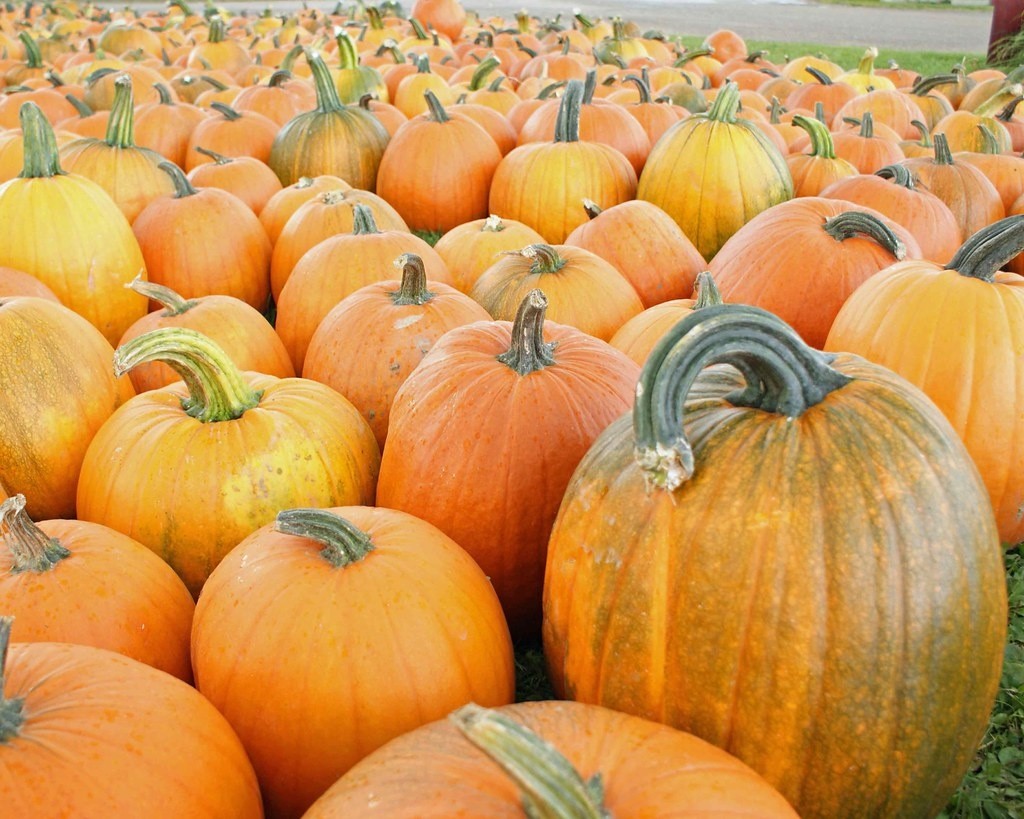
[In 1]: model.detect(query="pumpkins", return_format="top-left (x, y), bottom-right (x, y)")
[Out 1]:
top-left (0, 0), bottom-right (1024, 819)
